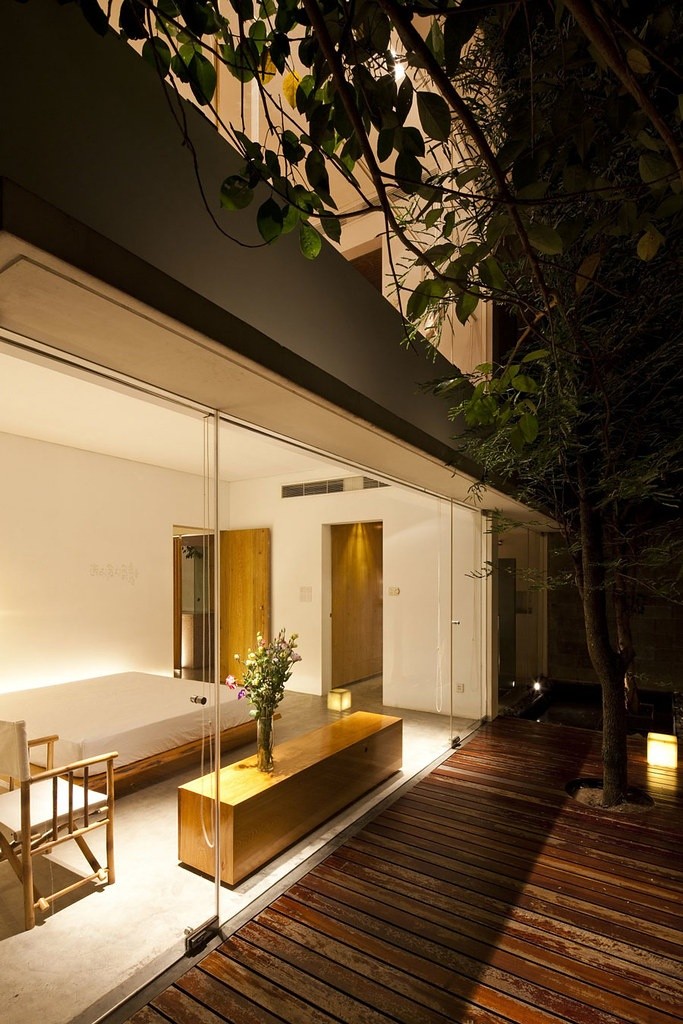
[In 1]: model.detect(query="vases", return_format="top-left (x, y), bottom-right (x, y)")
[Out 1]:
top-left (257, 716), bottom-right (274, 771)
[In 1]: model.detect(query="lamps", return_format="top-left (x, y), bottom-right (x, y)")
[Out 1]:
top-left (327, 688), bottom-right (352, 712)
top-left (646, 731), bottom-right (679, 770)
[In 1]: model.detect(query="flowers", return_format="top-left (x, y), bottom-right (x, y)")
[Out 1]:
top-left (226, 627), bottom-right (302, 769)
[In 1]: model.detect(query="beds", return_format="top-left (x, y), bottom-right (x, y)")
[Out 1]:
top-left (0, 670), bottom-right (257, 778)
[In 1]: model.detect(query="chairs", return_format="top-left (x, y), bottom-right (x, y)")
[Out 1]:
top-left (0, 720), bottom-right (118, 931)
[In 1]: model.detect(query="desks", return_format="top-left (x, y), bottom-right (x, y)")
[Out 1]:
top-left (177, 711), bottom-right (403, 885)
